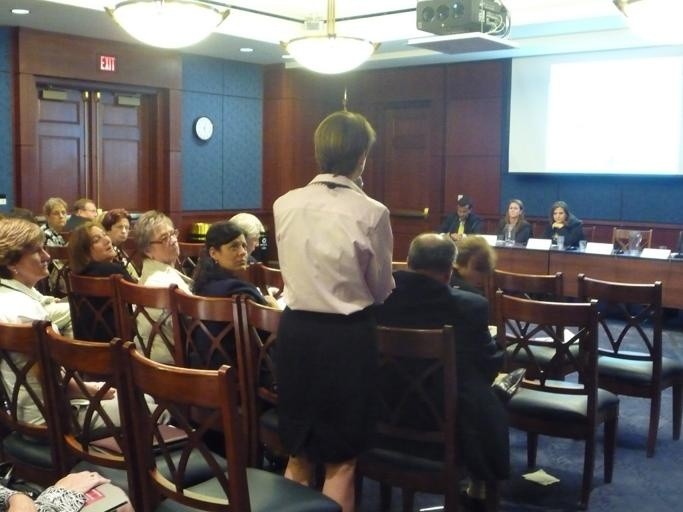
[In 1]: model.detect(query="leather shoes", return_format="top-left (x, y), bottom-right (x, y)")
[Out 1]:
top-left (489, 366), bottom-right (530, 404)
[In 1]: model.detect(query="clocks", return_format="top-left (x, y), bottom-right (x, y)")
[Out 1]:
top-left (192, 115), bottom-right (214, 143)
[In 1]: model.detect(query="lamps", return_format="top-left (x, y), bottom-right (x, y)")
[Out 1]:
top-left (284, 1), bottom-right (376, 77)
top-left (104, 1), bottom-right (230, 50)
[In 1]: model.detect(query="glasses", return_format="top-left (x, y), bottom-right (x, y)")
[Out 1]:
top-left (148, 228), bottom-right (179, 247)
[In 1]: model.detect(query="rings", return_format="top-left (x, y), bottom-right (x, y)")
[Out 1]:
top-left (90, 474), bottom-right (95, 478)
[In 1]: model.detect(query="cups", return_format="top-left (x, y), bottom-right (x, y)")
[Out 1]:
top-left (579, 241), bottom-right (587, 251)
top-left (557, 236), bottom-right (565, 251)
top-left (628, 231), bottom-right (642, 255)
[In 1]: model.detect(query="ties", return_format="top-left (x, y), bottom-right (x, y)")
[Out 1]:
top-left (457, 223), bottom-right (464, 234)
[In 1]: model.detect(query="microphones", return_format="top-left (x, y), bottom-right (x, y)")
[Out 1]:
top-left (444, 221), bottom-right (683, 258)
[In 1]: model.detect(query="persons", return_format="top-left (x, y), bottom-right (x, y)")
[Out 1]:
top-left (544, 200), bottom-right (584, 248)
top-left (453, 234), bottom-right (498, 297)
top-left (439, 196), bottom-right (484, 241)
top-left (494, 198), bottom-right (531, 247)
top-left (0, 470), bottom-right (135, 512)
top-left (382, 232), bottom-right (510, 512)
top-left (273, 113), bottom-right (396, 512)
top-left (1, 198), bottom-right (273, 443)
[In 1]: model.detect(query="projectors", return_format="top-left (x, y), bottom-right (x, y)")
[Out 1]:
top-left (416, 0), bottom-right (511, 35)
